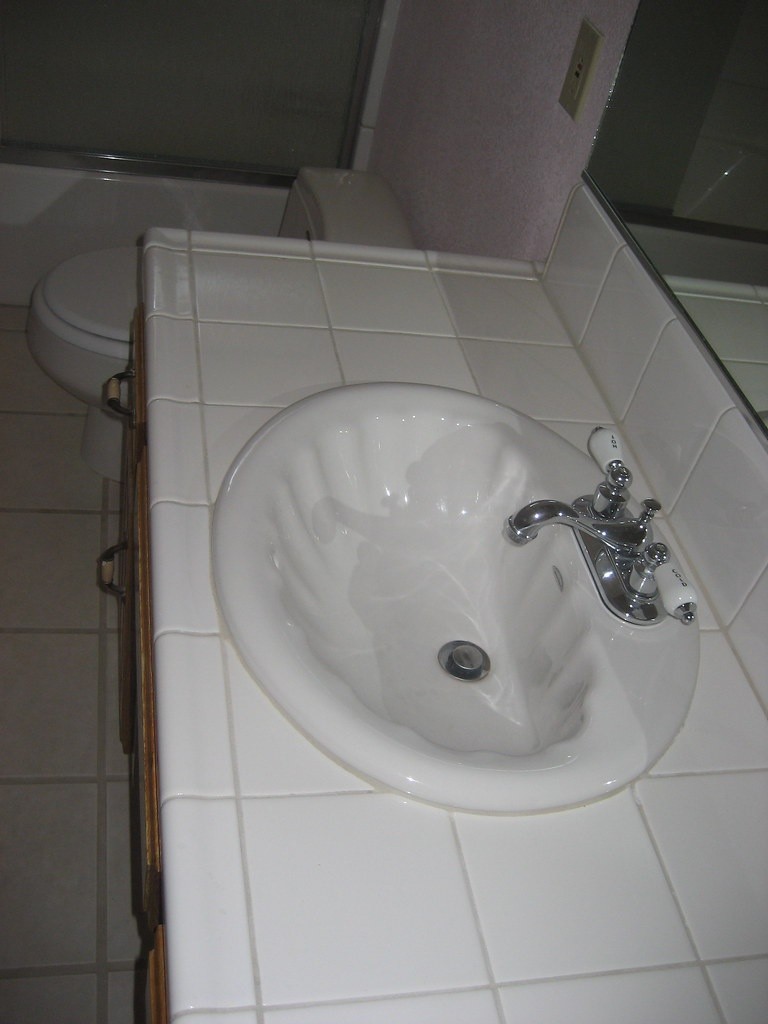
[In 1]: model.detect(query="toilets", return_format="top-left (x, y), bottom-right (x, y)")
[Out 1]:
top-left (24, 161), bottom-right (420, 414)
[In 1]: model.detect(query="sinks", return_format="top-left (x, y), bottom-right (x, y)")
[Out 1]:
top-left (203, 368), bottom-right (702, 821)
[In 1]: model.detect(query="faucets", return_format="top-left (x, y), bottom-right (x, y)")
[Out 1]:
top-left (505, 499), bottom-right (655, 546)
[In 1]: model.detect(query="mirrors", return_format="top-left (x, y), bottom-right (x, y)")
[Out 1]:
top-left (580, 0), bottom-right (768, 441)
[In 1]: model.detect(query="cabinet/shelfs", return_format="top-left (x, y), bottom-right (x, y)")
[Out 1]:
top-left (98, 304), bottom-right (168, 1024)
